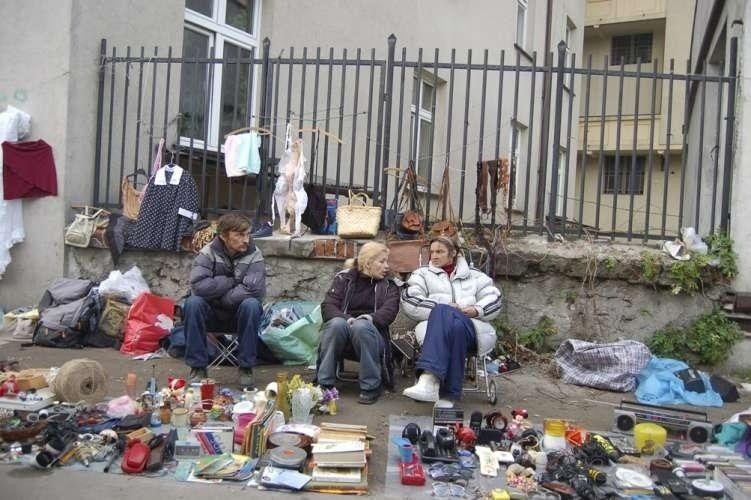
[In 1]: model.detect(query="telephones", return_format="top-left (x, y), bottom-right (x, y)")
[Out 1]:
top-left (122, 442), bottom-right (148, 473)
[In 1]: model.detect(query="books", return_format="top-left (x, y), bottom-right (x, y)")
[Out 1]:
top-left (304, 422), bottom-right (378, 491)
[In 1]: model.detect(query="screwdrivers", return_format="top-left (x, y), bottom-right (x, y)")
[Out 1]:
top-left (59, 444), bottom-right (83, 465)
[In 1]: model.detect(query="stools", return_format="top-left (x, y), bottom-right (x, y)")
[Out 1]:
top-left (204, 328), bottom-right (242, 374)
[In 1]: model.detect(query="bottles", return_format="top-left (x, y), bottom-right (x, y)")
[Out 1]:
top-left (170, 408), bottom-right (192, 441)
top-left (126, 373), bottom-right (138, 401)
top-left (150, 395), bottom-right (162, 433)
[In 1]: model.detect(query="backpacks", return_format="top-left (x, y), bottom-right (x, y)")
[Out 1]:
top-left (64, 206), bottom-right (103, 248)
top-left (21, 299), bottom-right (97, 349)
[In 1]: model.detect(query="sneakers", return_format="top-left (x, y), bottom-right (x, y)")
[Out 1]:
top-left (402, 373), bottom-right (440, 402)
top-left (358, 386), bottom-right (379, 403)
top-left (433, 399), bottom-right (456, 409)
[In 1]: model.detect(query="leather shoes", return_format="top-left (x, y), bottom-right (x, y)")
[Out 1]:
top-left (187, 368), bottom-right (206, 387)
top-left (236, 367), bottom-right (255, 390)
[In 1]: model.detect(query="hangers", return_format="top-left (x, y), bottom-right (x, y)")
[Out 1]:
top-left (124, 151), bottom-right (188, 186)
top-left (2, 122), bottom-right (49, 147)
top-left (382, 158), bottom-right (467, 187)
top-left (223, 111), bottom-right (346, 147)
top-left (71, 202), bottom-right (112, 215)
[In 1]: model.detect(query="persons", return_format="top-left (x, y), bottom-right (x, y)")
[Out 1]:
top-left (398, 236), bottom-right (505, 408)
top-left (181, 212), bottom-right (267, 392)
top-left (313, 240), bottom-right (402, 404)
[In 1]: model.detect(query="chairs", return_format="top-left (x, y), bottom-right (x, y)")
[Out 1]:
top-left (410, 245), bottom-right (498, 406)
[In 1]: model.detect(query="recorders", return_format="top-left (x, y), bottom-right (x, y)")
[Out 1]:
top-left (612, 400), bottom-right (713, 447)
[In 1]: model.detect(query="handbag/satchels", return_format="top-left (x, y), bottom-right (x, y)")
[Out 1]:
top-left (335, 189), bottom-right (381, 238)
top-left (432, 221), bottom-right (455, 239)
top-left (258, 300), bottom-right (322, 367)
top-left (547, 337), bottom-right (652, 393)
top-left (384, 209), bottom-right (422, 240)
